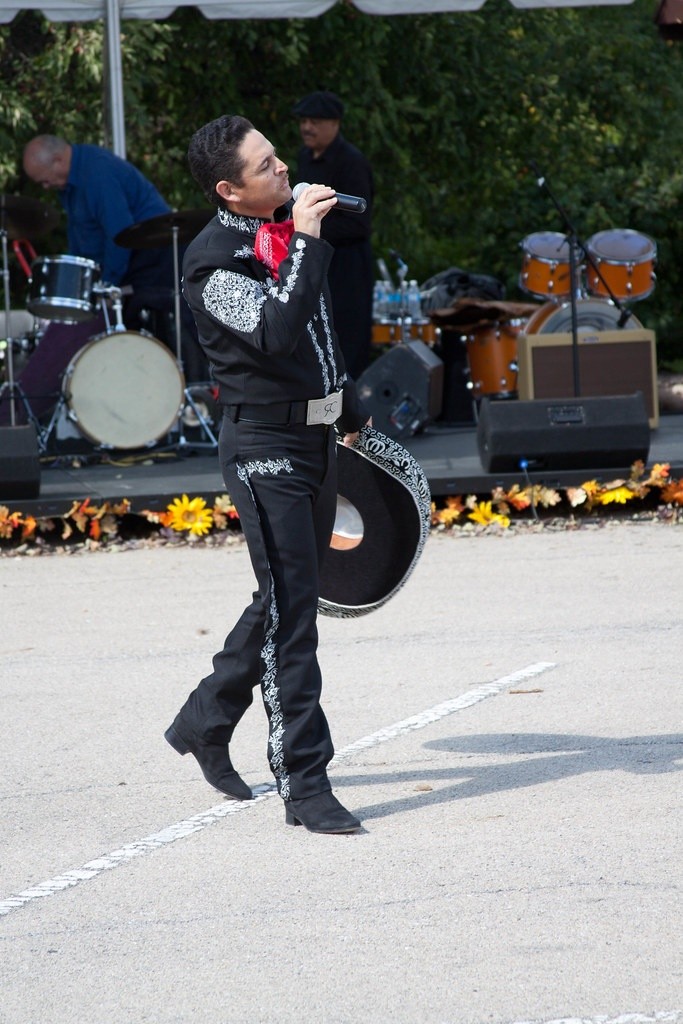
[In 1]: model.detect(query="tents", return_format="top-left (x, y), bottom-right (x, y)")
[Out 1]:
top-left (0, 1), bottom-right (633, 162)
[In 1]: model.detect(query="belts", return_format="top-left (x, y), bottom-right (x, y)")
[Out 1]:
top-left (221, 389), bottom-right (343, 426)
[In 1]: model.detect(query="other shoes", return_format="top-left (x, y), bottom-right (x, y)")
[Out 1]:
top-left (285, 789), bottom-right (361, 833)
top-left (164, 711), bottom-right (253, 800)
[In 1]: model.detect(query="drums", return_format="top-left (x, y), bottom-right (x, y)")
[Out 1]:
top-left (523, 296), bottom-right (645, 334)
top-left (60, 328), bottom-right (186, 452)
top-left (585, 227), bottom-right (659, 303)
top-left (25, 252), bottom-right (103, 326)
top-left (519, 230), bottom-right (585, 301)
top-left (372, 316), bottom-right (437, 349)
top-left (465, 317), bottom-right (531, 400)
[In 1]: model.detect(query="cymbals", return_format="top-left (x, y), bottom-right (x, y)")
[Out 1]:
top-left (0, 194), bottom-right (62, 242)
top-left (112, 206), bottom-right (219, 250)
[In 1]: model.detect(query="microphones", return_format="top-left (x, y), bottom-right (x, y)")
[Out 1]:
top-left (291, 183), bottom-right (366, 213)
top-left (617, 308), bottom-right (633, 328)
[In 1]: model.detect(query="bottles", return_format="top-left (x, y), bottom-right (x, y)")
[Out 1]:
top-left (372, 280), bottom-right (421, 321)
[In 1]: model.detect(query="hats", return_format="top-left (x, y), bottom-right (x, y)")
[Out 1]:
top-left (293, 92), bottom-right (343, 119)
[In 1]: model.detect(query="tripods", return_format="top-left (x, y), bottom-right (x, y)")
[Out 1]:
top-left (0, 230), bottom-right (48, 457)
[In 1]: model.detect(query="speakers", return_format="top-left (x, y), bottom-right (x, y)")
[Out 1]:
top-left (0, 426), bottom-right (41, 500)
top-left (352, 337), bottom-right (443, 439)
top-left (476, 398), bottom-right (651, 474)
top-left (517, 328), bottom-right (659, 429)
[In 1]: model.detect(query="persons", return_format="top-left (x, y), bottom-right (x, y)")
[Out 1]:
top-left (24, 136), bottom-right (173, 339)
top-left (288, 91), bottom-right (374, 370)
top-left (163, 118), bottom-right (361, 834)
